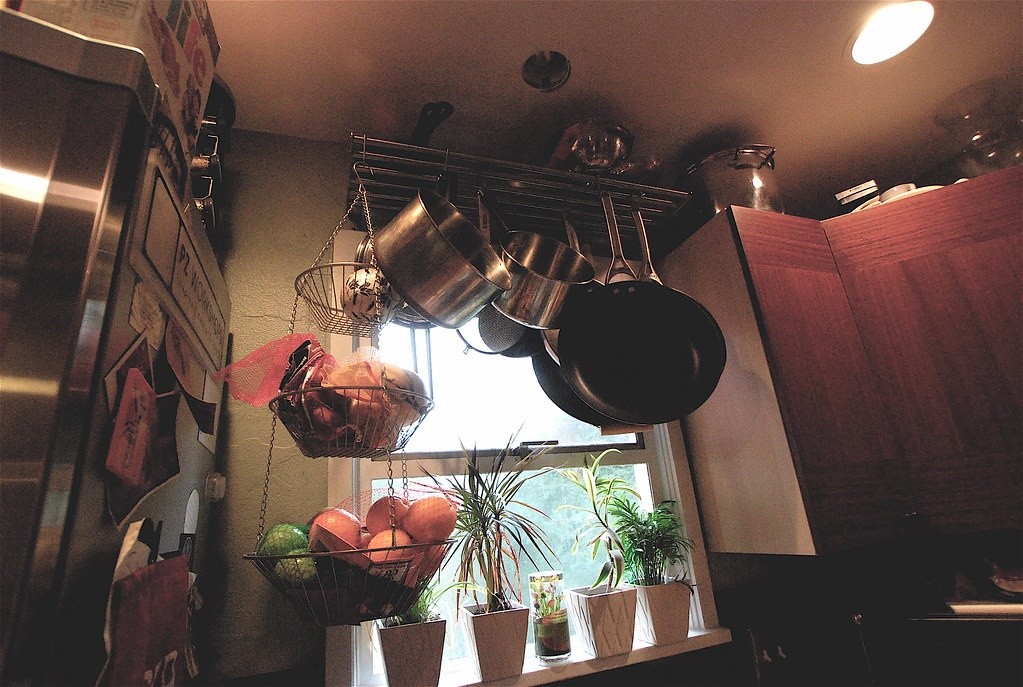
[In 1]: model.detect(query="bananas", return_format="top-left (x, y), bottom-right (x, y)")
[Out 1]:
top-left (383, 364), bottom-right (428, 415)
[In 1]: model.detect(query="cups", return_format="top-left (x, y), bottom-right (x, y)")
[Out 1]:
top-left (528, 570), bottom-right (572, 667)
top-left (880, 183), bottom-right (916, 202)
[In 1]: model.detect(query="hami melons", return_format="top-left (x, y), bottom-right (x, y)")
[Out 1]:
top-left (386, 370), bottom-right (428, 425)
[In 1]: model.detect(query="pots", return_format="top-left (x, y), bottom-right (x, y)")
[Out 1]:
top-left (373, 169), bottom-right (728, 428)
top-left (687, 143), bottom-right (784, 216)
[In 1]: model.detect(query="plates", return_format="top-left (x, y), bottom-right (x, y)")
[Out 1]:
top-left (883, 186), bottom-right (945, 203)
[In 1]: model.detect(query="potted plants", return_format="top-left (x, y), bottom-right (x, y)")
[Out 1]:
top-left (373, 580), bottom-right (488, 687)
top-left (539, 449), bottom-right (637, 660)
top-left (607, 498), bottom-right (698, 647)
top-left (409, 421), bottom-right (529, 683)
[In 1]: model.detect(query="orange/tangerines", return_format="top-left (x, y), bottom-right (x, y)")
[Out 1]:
top-left (256, 495), bottom-right (456, 588)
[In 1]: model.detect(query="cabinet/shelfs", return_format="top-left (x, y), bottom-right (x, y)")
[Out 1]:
top-left (652, 164), bottom-right (1023, 561)
